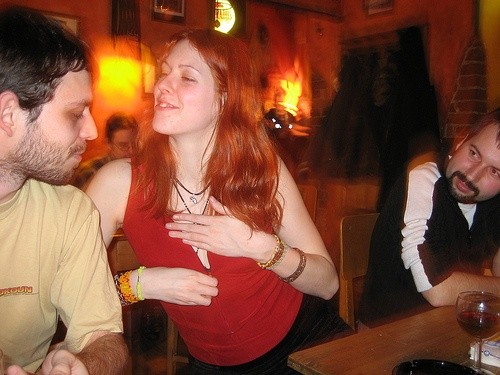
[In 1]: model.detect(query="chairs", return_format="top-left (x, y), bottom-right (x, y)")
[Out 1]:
top-left (167, 186), bottom-right (318, 375)
top-left (339, 211), bottom-right (378, 329)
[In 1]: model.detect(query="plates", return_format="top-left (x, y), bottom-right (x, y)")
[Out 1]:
top-left (391, 359), bottom-right (480, 375)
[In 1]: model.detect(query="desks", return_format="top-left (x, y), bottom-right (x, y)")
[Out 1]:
top-left (288, 307), bottom-right (500, 375)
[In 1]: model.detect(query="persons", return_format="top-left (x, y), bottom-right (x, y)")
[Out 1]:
top-left (0, 6), bottom-right (130, 375)
top-left (70, 42), bottom-right (439, 192)
top-left (83, 28), bottom-right (357, 374)
top-left (357, 106), bottom-right (500, 332)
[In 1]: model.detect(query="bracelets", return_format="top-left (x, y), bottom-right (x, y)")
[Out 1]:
top-left (256, 231), bottom-right (308, 285)
top-left (113, 264), bottom-right (146, 308)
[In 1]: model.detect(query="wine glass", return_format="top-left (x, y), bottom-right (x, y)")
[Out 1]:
top-left (455, 290), bottom-right (500, 375)
